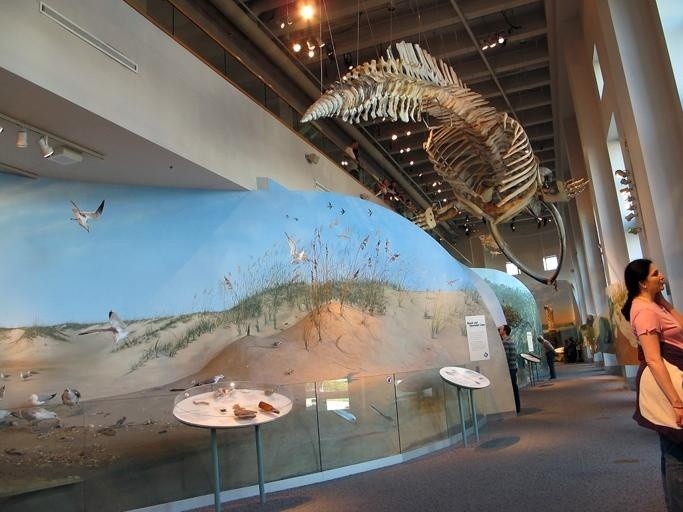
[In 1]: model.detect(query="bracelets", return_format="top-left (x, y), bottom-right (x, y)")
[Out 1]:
top-left (672, 404), bottom-right (683, 409)
top-left (663, 302), bottom-right (672, 310)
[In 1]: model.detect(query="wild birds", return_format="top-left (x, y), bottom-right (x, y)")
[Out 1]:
top-left (223, 277), bottom-right (233, 291)
top-left (285, 214), bottom-right (298, 221)
top-left (283, 231), bottom-right (310, 266)
top-left (68, 198), bottom-right (105, 233)
top-left (78, 310), bottom-right (137, 351)
top-left (194, 374), bottom-right (225, 387)
top-left (0, 370), bottom-right (83, 430)
top-left (328, 199), bottom-right (372, 216)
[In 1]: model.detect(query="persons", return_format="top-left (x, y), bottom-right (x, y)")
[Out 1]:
top-left (371, 176), bottom-right (456, 256)
top-left (496, 324), bottom-right (522, 413)
top-left (562, 335), bottom-right (583, 363)
top-left (341, 138), bottom-right (362, 182)
top-left (536, 334), bottom-right (561, 379)
top-left (619, 257), bottom-right (682, 511)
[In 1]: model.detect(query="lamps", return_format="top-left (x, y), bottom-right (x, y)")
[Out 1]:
top-left (276, 0), bottom-right (552, 244)
top-left (0, 113), bottom-right (55, 159)
